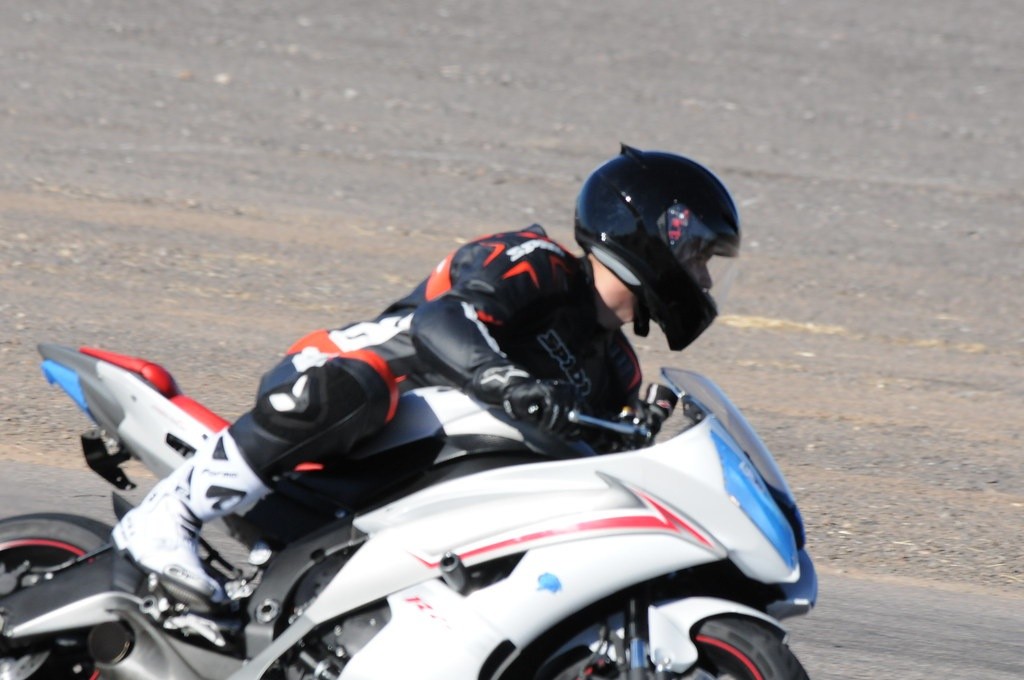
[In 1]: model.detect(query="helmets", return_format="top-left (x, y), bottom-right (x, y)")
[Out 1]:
top-left (574, 141), bottom-right (741, 350)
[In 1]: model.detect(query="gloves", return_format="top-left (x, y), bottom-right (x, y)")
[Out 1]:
top-left (502, 378), bottom-right (584, 441)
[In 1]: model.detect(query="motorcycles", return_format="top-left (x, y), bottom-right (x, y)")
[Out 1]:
top-left (1, 338), bottom-right (818, 680)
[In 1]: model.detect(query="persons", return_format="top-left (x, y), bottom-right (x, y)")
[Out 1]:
top-left (110, 144), bottom-right (742, 618)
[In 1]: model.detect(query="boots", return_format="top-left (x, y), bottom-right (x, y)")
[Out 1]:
top-left (112, 427), bottom-right (274, 614)
